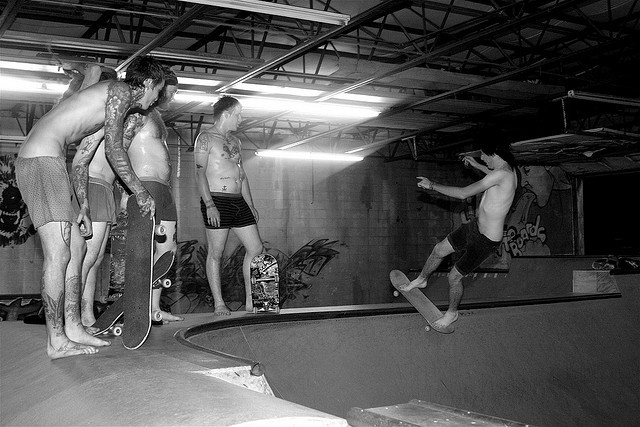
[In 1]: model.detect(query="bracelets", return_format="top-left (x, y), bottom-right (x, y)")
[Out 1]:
top-left (205, 199), bottom-right (215, 208)
top-left (429, 181), bottom-right (434, 191)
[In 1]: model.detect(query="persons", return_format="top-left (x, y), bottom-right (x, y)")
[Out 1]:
top-left (194, 95), bottom-right (263, 316)
top-left (398, 144), bottom-right (518, 330)
top-left (14, 55), bottom-right (165, 360)
top-left (70, 124), bottom-right (117, 328)
top-left (120, 65), bottom-right (185, 325)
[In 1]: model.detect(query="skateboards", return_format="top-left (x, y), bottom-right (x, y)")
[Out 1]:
top-left (388, 269), bottom-right (456, 335)
top-left (121, 194), bottom-right (165, 351)
top-left (90, 251), bottom-right (177, 337)
top-left (248, 252), bottom-right (281, 315)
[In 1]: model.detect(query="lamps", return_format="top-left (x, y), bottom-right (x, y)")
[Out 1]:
top-left (178, 0), bottom-right (351, 27)
